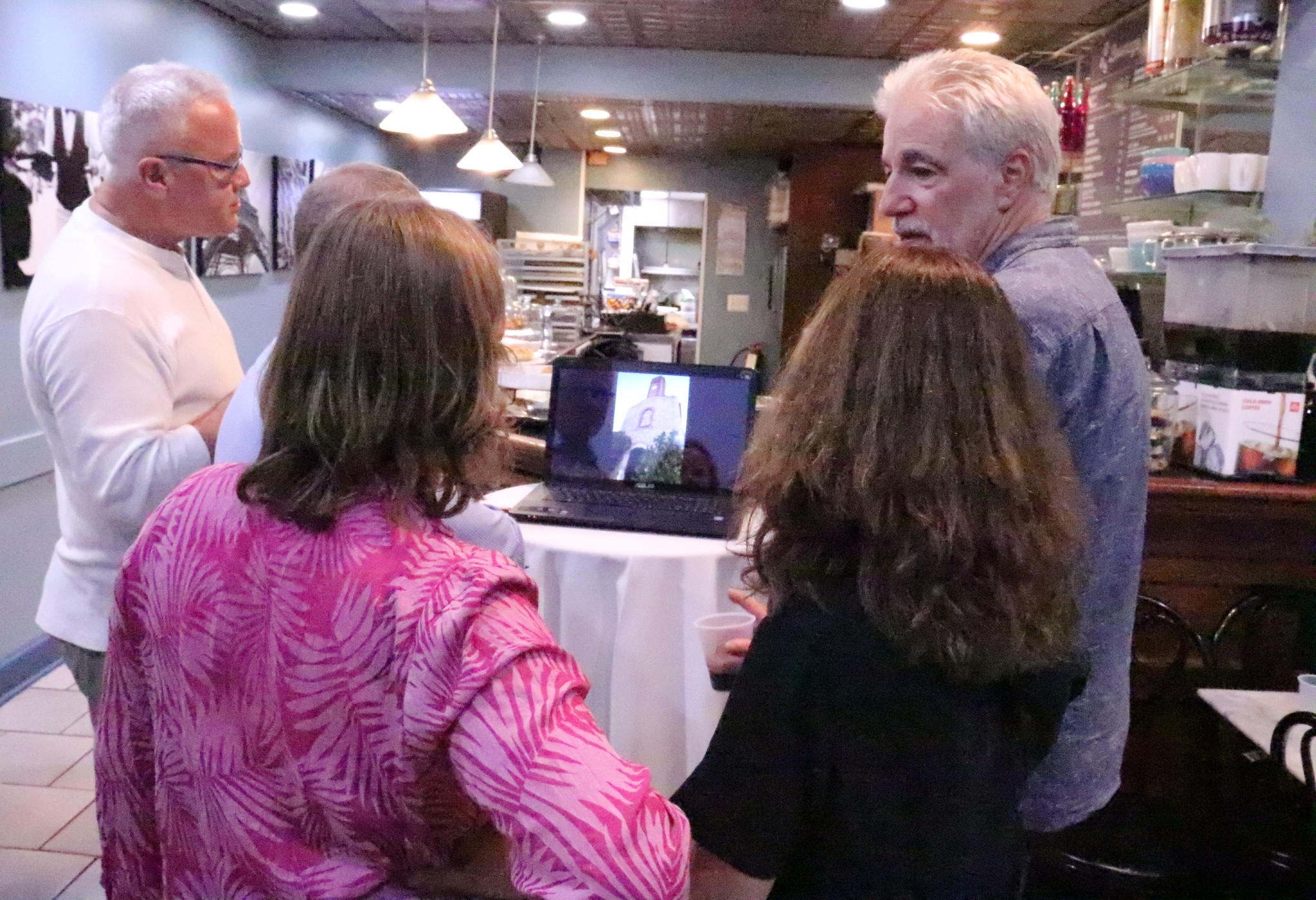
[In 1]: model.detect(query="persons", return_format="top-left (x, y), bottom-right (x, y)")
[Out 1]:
top-left (876, 49), bottom-right (1153, 834)
top-left (93, 192), bottom-right (693, 900)
top-left (18, 61), bottom-right (250, 715)
top-left (213, 165), bottom-right (529, 568)
top-left (683, 243), bottom-right (1095, 900)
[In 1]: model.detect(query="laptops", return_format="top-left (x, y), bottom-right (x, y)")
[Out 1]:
top-left (504, 356), bottom-right (758, 539)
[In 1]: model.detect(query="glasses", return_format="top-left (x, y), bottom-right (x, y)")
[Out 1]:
top-left (150, 142), bottom-right (246, 185)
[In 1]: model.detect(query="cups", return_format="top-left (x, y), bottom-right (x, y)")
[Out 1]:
top-left (1142, 232), bottom-right (1252, 272)
top-left (1172, 150), bottom-right (1268, 193)
top-left (694, 612), bottom-right (756, 691)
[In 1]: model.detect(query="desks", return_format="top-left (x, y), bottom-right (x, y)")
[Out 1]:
top-left (1132, 472), bottom-right (1316, 693)
top-left (468, 479), bottom-right (784, 801)
top-left (1194, 684), bottom-right (1316, 791)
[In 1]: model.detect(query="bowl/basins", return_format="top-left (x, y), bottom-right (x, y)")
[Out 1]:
top-left (1138, 146), bottom-right (1190, 195)
top-left (1106, 220), bottom-right (1174, 273)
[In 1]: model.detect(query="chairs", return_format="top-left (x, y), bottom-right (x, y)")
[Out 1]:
top-left (1057, 590), bottom-right (1316, 900)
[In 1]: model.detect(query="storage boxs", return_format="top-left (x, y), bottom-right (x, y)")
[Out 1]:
top-left (1160, 238), bottom-right (1316, 336)
top-left (1169, 358), bottom-right (1308, 483)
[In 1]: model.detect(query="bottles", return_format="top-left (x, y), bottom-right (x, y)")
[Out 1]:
top-left (1043, 74), bottom-right (1090, 152)
top-left (1143, 356), bottom-right (1180, 474)
top-left (1056, 172), bottom-right (1081, 215)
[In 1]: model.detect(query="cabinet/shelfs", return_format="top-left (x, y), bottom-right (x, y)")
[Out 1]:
top-left (1104, 1), bottom-right (1289, 220)
top-left (496, 228), bottom-right (590, 362)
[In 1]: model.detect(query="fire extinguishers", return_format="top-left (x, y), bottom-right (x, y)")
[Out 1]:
top-left (729, 343), bottom-right (763, 396)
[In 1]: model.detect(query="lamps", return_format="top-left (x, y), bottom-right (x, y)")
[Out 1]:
top-left (376, 0), bottom-right (469, 144)
top-left (455, 0), bottom-right (524, 177)
top-left (503, 34), bottom-right (559, 191)
